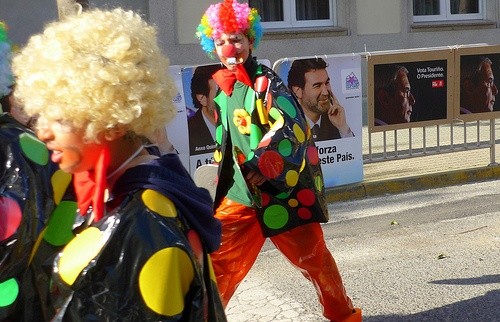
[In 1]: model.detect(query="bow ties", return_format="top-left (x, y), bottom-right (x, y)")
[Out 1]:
top-left (74, 144), bottom-right (112, 223)
top-left (211, 63), bottom-right (252, 97)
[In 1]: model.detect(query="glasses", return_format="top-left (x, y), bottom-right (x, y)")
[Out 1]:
top-left (395, 88), bottom-right (410, 97)
top-left (478, 80), bottom-right (495, 88)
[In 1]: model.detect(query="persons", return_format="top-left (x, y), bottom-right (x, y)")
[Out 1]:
top-left (460, 55), bottom-right (498, 114)
top-left (287, 58), bottom-right (355, 142)
top-left (196, 0), bottom-right (362, 322)
top-left (187, 63), bottom-right (228, 156)
top-left (0, 3), bottom-right (227, 322)
top-left (374, 64), bottom-right (416, 126)
